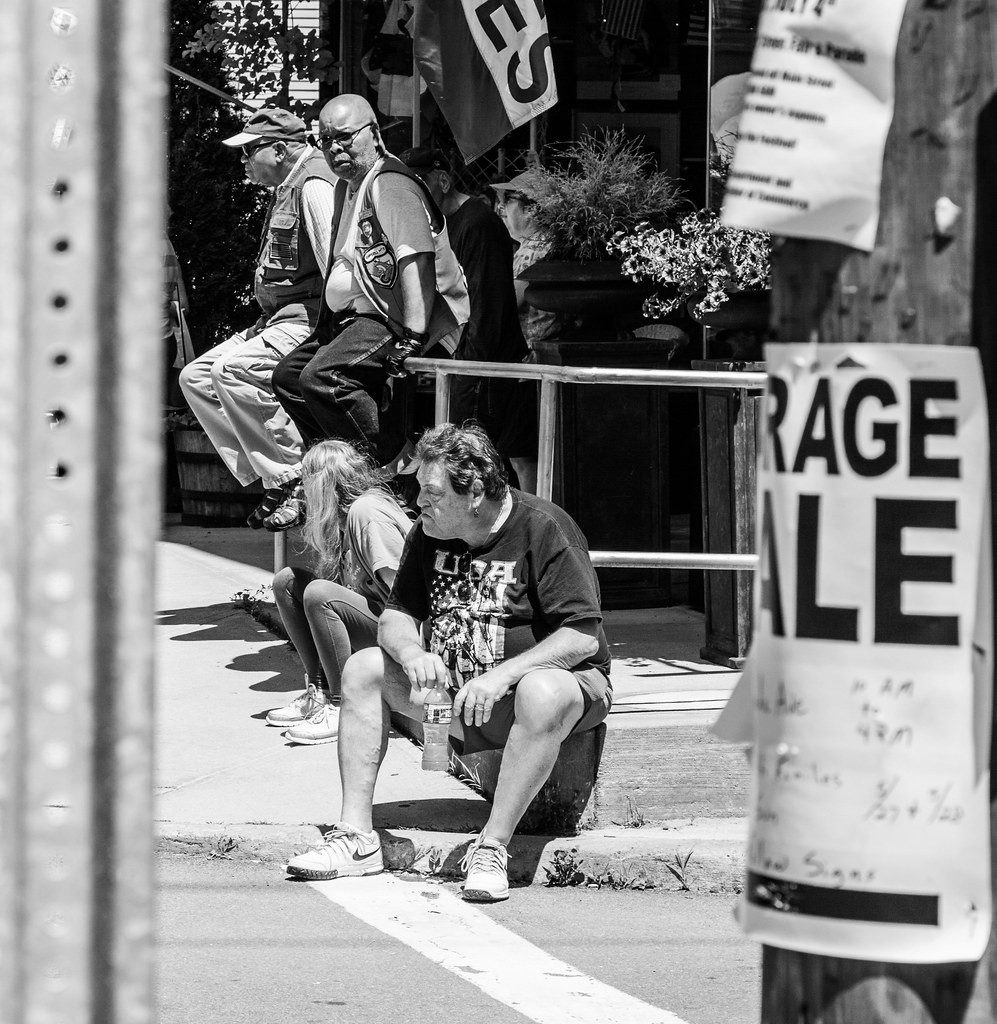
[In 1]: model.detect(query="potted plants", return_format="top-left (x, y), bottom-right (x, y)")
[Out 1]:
top-left (603, 129), bottom-right (773, 361)
top-left (163, 0), bottom-right (347, 528)
top-left (514, 122), bottom-right (698, 337)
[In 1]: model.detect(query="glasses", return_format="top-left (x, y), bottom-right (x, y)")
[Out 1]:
top-left (502, 194), bottom-right (524, 202)
top-left (457, 550), bottom-right (472, 604)
top-left (242, 138), bottom-right (288, 158)
top-left (315, 122), bottom-right (374, 151)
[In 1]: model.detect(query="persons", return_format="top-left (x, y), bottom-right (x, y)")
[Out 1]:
top-left (162, 232), bottom-right (187, 374)
top-left (178, 103), bottom-right (342, 529)
top-left (287, 424), bottom-right (612, 901)
top-left (272, 93), bottom-right (469, 481)
top-left (267, 441), bottom-right (415, 745)
top-left (396, 144), bottom-right (560, 496)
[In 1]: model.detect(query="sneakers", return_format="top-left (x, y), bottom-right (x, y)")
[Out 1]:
top-left (457, 828), bottom-right (512, 900)
top-left (266, 683), bottom-right (328, 727)
top-left (286, 704), bottom-right (341, 746)
top-left (287, 822), bottom-right (385, 880)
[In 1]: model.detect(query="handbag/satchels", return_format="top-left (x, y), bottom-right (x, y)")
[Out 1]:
top-left (170, 282), bottom-right (194, 368)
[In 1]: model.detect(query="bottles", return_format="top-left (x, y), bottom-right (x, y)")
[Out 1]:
top-left (421, 675), bottom-right (452, 773)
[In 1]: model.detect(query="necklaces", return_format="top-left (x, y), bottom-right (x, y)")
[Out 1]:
top-left (350, 174), bottom-right (367, 195)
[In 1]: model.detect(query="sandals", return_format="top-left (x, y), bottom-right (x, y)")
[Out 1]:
top-left (246, 481), bottom-right (304, 532)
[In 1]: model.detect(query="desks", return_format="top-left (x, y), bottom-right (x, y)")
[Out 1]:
top-left (401, 355), bottom-right (769, 668)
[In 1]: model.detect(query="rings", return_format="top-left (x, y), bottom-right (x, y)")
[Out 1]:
top-left (476, 703), bottom-right (484, 706)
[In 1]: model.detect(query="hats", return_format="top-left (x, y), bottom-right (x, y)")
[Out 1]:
top-left (398, 146), bottom-right (451, 178)
top-left (221, 109), bottom-right (306, 146)
top-left (489, 169), bottom-right (561, 216)
